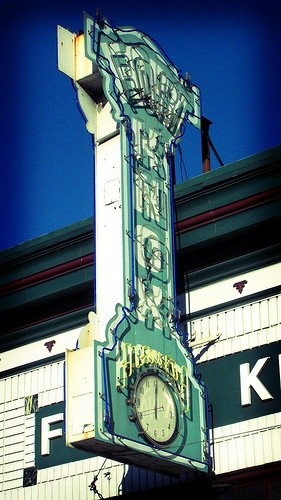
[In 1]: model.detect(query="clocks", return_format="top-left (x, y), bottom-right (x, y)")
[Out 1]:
top-left (123, 360), bottom-right (185, 448)
